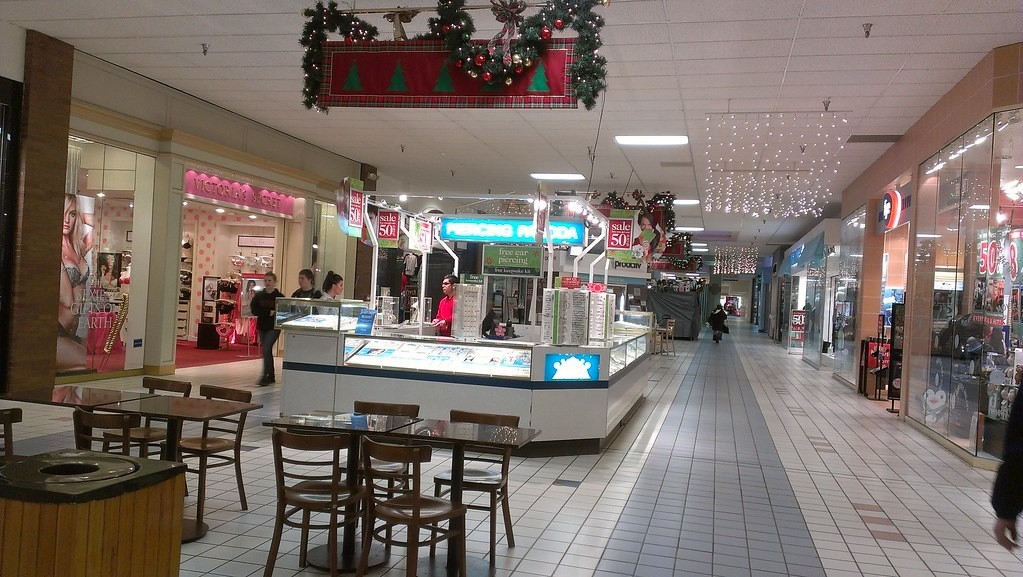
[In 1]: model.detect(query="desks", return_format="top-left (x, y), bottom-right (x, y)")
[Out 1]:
top-left (51, 386), bottom-right (161, 451)
top-left (94, 395), bottom-right (263, 544)
top-left (262, 410), bottom-right (424, 573)
top-left (388, 419), bottom-right (542, 577)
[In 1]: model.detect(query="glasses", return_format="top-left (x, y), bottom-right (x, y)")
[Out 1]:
top-left (441, 282), bottom-right (452, 285)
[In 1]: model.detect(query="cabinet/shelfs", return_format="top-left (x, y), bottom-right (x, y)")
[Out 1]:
top-left (176, 299), bottom-right (191, 341)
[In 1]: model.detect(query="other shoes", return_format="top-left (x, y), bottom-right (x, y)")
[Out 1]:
top-left (257, 374), bottom-right (275, 385)
top-left (260, 373), bottom-right (272, 386)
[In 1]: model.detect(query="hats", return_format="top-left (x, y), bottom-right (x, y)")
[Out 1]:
top-left (717, 304), bottom-right (724, 307)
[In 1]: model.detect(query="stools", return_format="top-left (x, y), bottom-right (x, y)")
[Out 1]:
top-left (653, 318), bottom-right (676, 357)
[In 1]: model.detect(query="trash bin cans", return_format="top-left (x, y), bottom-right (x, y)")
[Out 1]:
top-left (0, 447), bottom-right (188, 577)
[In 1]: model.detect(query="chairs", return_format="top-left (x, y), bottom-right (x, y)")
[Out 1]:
top-left (356, 435), bottom-right (467, 577)
top-left (429, 409), bottom-right (521, 568)
top-left (102, 377), bottom-right (193, 497)
top-left (338, 400), bottom-right (421, 550)
top-left (0, 407), bottom-right (30, 469)
top-left (263, 424), bottom-right (370, 577)
top-left (180, 384), bottom-right (252, 521)
top-left (72, 406), bottom-right (141, 456)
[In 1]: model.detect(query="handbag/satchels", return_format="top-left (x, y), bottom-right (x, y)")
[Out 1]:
top-left (722, 325), bottom-right (730, 334)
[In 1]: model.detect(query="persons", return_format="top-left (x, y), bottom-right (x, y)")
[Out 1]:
top-left (633, 209), bottom-right (666, 253)
top-left (242, 280), bottom-right (258, 316)
top-left (119, 266), bottom-right (131, 299)
top-left (990, 376), bottom-right (1023, 551)
top-left (400, 270), bottom-right (408, 322)
top-left (316, 270), bottom-right (343, 315)
top-left (56, 194), bottom-right (94, 370)
top-left (100, 254), bottom-right (117, 289)
top-left (706, 303), bottom-right (727, 344)
top-left (250, 271), bottom-right (288, 386)
top-left (291, 269), bottom-right (322, 315)
top-left (431, 274), bottom-right (460, 337)
top-left (181, 233), bottom-right (194, 262)
